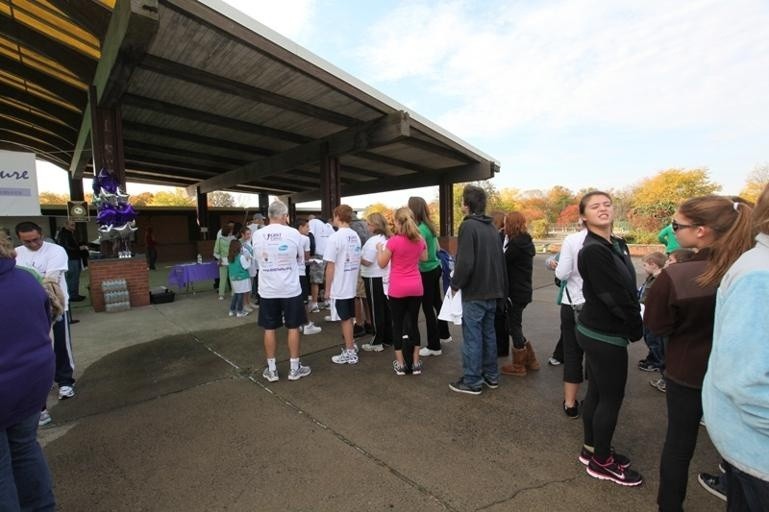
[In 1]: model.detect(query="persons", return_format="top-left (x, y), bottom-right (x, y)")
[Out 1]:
top-left (500, 210), bottom-right (542, 376)
top-left (212, 186), bottom-right (454, 381)
top-left (545, 190), bottom-right (644, 486)
top-left (638, 185), bottom-right (769, 512)
top-left (145, 226), bottom-right (161, 271)
top-left (40, 229), bottom-right (54, 244)
top-left (0, 233), bottom-right (56, 511)
top-left (487, 210), bottom-right (512, 361)
top-left (78, 231), bottom-right (89, 271)
top-left (56, 220), bottom-right (87, 303)
top-left (12, 221), bottom-right (78, 401)
top-left (448, 185), bottom-right (509, 395)
top-left (0, 227), bottom-right (52, 427)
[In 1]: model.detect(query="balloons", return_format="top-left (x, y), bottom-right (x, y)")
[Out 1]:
top-left (92, 168), bottom-right (139, 245)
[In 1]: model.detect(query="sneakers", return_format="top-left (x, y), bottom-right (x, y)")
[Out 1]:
top-left (218, 296), bottom-right (225, 300)
top-left (60, 382), bottom-right (76, 400)
top-left (480, 371), bottom-right (498, 389)
top-left (331, 350), bottom-right (358, 364)
top-left (287, 366), bottom-right (312, 381)
top-left (448, 379), bottom-right (482, 394)
top-left (353, 321), bottom-right (390, 351)
top-left (419, 337), bottom-right (452, 357)
top-left (229, 299), bottom-right (260, 318)
top-left (698, 464), bottom-right (730, 502)
top-left (263, 368), bottom-right (279, 382)
top-left (580, 445), bottom-right (643, 487)
top-left (38, 410), bottom-right (51, 426)
top-left (639, 356), bottom-right (667, 392)
top-left (70, 295), bottom-right (85, 301)
top-left (392, 359), bottom-right (423, 376)
top-left (549, 356), bottom-right (560, 366)
top-left (563, 398), bottom-right (579, 418)
top-left (303, 323), bottom-right (322, 335)
top-left (309, 302), bottom-right (333, 322)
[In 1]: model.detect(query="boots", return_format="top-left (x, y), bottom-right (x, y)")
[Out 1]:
top-left (525, 341), bottom-right (540, 370)
top-left (501, 340), bottom-right (528, 377)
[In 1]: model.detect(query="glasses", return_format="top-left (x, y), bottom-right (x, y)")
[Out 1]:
top-left (673, 223), bottom-right (695, 232)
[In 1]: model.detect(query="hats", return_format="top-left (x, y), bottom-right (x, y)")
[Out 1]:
top-left (253, 214), bottom-right (266, 219)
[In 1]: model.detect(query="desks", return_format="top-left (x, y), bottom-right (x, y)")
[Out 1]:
top-left (169, 262), bottom-right (219, 297)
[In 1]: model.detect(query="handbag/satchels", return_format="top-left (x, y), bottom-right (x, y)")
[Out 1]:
top-left (217, 259), bottom-right (221, 268)
